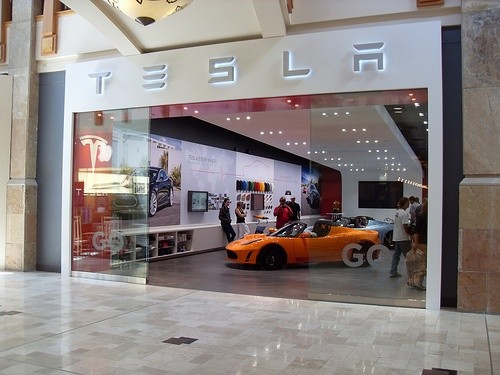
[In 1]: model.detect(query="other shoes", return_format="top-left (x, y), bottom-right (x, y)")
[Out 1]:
top-left (389, 271), bottom-right (402, 277)
top-left (413, 284), bottom-right (426, 291)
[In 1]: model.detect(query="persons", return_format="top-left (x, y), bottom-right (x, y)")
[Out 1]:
top-left (406, 198), bottom-right (428, 290)
top-left (286, 197), bottom-right (301, 222)
top-left (274, 196), bottom-right (293, 229)
top-left (409, 196), bottom-right (421, 240)
top-left (235, 202), bottom-right (250, 239)
top-left (219, 198), bottom-right (236, 248)
top-left (389, 197), bottom-right (414, 277)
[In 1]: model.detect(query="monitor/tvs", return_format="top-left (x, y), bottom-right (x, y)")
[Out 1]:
top-left (251, 194), bottom-right (264, 210)
top-left (358, 181), bottom-right (403, 208)
top-left (188, 191), bottom-right (208, 212)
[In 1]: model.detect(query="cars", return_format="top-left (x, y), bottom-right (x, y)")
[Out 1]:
top-left (109, 166), bottom-right (174, 217)
top-left (305, 183), bottom-right (319, 208)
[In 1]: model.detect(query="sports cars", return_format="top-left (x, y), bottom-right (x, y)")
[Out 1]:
top-left (223, 220), bottom-right (381, 267)
top-left (333, 215), bottom-right (396, 249)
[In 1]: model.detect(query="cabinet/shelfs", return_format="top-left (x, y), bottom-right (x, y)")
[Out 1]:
top-left (111, 214), bottom-right (325, 268)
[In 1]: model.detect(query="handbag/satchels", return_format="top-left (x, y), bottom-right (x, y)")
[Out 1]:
top-left (406, 225), bottom-right (416, 234)
